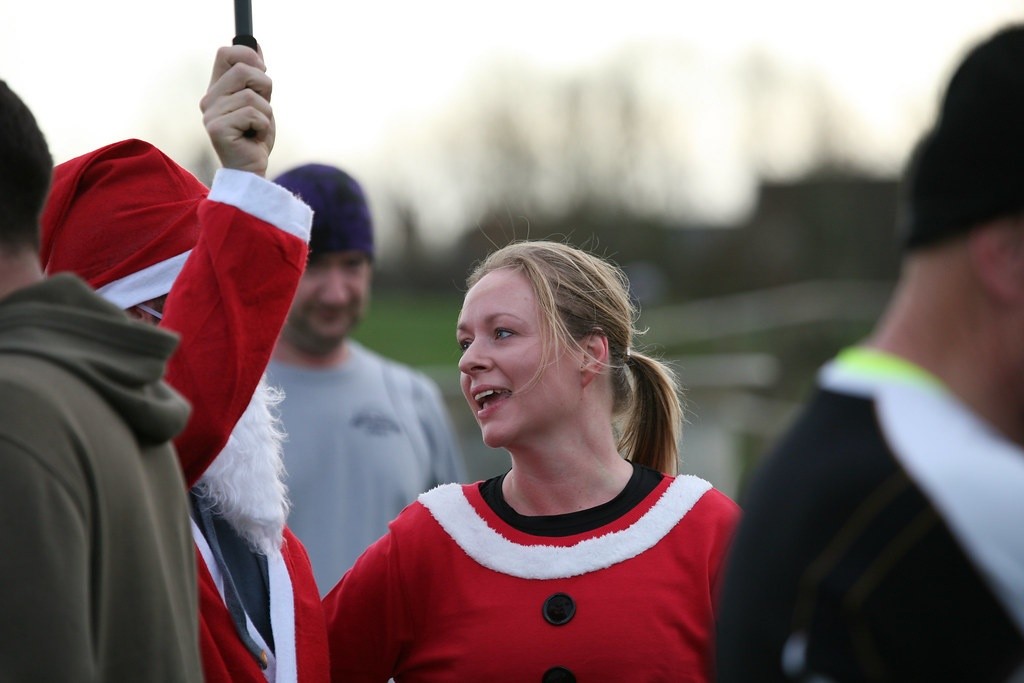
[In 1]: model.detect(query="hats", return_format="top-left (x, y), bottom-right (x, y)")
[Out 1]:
top-left (898, 16), bottom-right (1024, 249)
top-left (41, 141), bottom-right (212, 312)
top-left (274, 162), bottom-right (373, 262)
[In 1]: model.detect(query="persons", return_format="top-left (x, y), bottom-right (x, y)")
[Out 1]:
top-left (0, 80), bottom-right (204, 683)
top-left (266, 162), bottom-right (470, 601)
top-left (715, 19), bottom-right (1024, 683)
top-left (324, 197), bottom-right (745, 683)
top-left (38, 41), bottom-right (331, 683)
